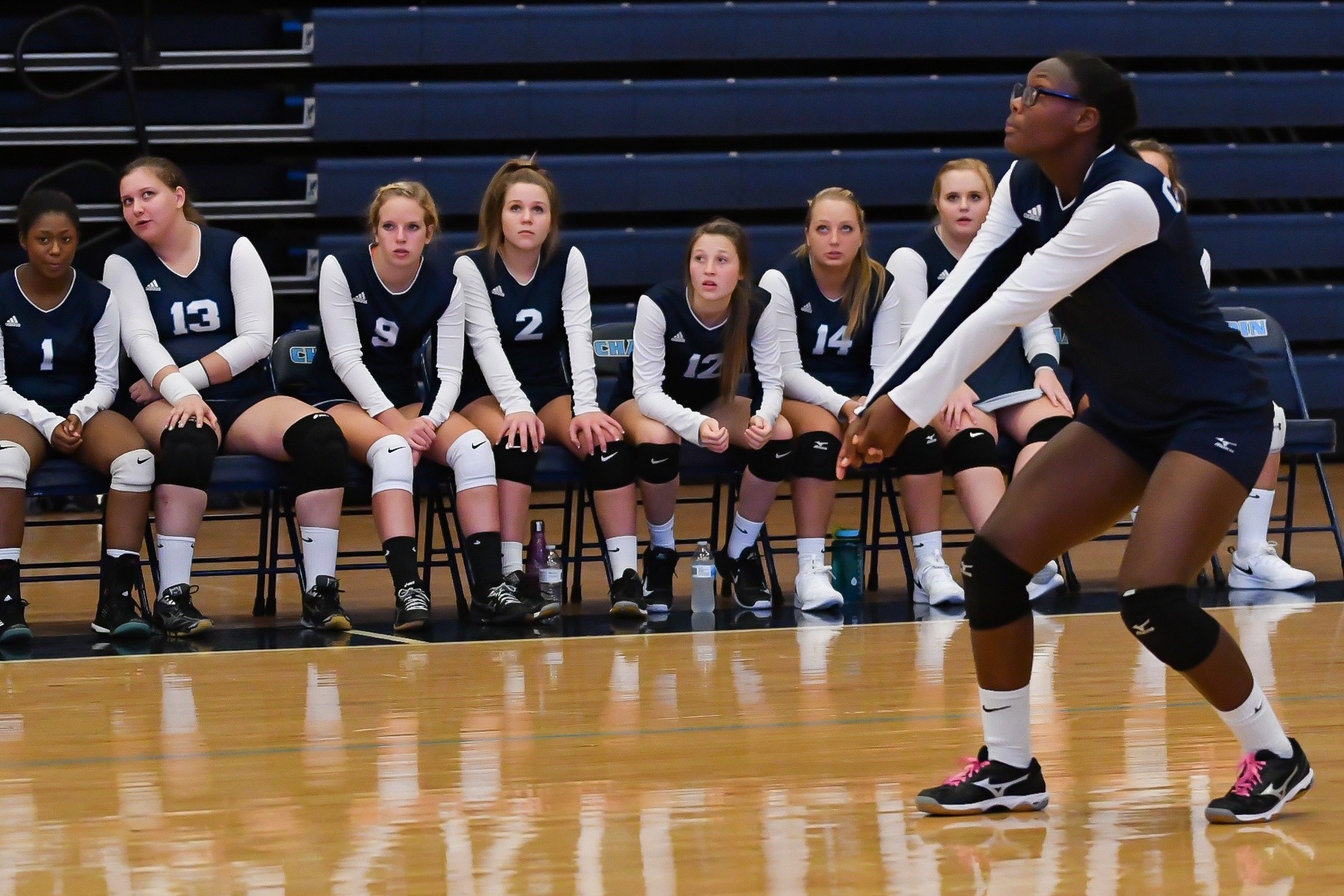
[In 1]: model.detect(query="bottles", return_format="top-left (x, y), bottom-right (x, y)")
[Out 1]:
top-left (539, 545), bottom-right (563, 617)
top-left (831, 527), bottom-right (864, 602)
top-left (690, 541), bottom-right (715, 613)
top-left (525, 519), bottom-right (548, 595)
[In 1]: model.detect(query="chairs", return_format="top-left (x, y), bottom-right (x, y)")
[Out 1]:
top-left (19, 307), bottom-right (1344, 634)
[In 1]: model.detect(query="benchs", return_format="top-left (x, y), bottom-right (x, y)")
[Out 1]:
top-left (303, 0), bottom-right (1344, 437)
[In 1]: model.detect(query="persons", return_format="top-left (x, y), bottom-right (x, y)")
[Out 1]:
top-left (606, 218), bottom-right (792, 610)
top-left (1074, 139), bottom-right (1318, 591)
top-left (101, 155), bottom-right (353, 637)
top-left (454, 153), bottom-right (650, 620)
top-left (0, 190), bottom-right (156, 648)
top-left (760, 187), bottom-right (967, 613)
top-left (885, 162), bottom-right (1076, 602)
top-left (836, 50), bottom-right (1319, 826)
top-left (305, 181), bottom-right (538, 630)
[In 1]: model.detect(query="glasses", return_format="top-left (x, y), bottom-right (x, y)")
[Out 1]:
top-left (1009, 79), bottom-right (1100, 110)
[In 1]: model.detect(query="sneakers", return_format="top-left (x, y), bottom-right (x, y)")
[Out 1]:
top-left (470, 582), bottom-right (534, 623)
top-left (90, 590), bottom-right (152, 636)
top-left (1025, 560), bottom-right (1064, 601)
top-left (151, 583), bottom-right (214, 636)
top-left (394, 578), bottom-right (431, 631)
top-left (503, 569), bottom-right (562, 619)
top-left (1203, 737), bottom-right (1314, 823)
top-left (714, 542), bottom-right (773, 609)
top-left (301, 573), bottom-right (352, 632)
top-left (640, 545), bottom-right (680, 613)
top-left (792, 565), bottom-right (844, 611)
top-left (915, 744), bottom-right (1050, 816)
top-left (0, 594), bottom-right (34, 643)
top-left (607, 568), bottom-right (648, 616)
top-left (913, 549), bottom-right (965, 606)
top-left (1226, 540), bottom-right (1316, 592)
